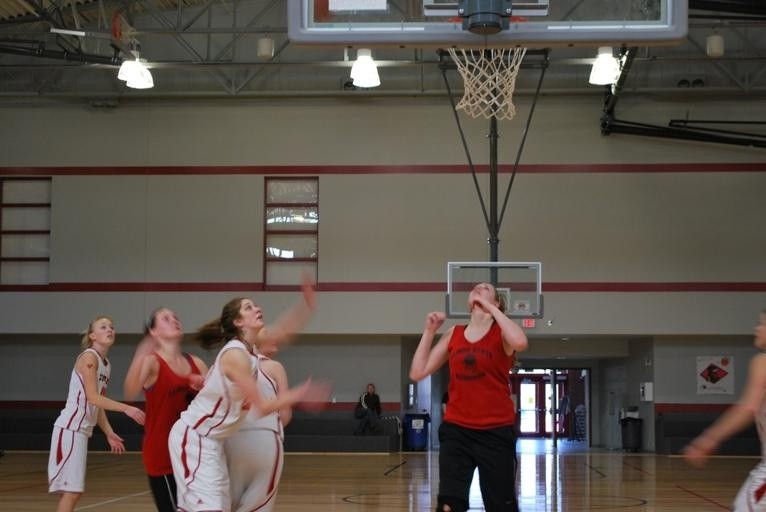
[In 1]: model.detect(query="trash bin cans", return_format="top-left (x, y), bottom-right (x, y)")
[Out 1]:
top-left (621, 418), bottom-right (643, 453)
top-left (401, 414), bottom-right (431, 452)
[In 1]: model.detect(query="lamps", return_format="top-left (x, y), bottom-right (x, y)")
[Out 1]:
top-left (116, 41), bottom-right (141, 81)
top-left (348, 47), bottom-right (381, 88)
top-left (124, 58), bottom-right (154, 89)
top-left (586, 46), bottom-right (621, 86)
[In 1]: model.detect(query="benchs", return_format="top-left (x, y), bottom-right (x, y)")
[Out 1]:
top-left (283, 413), bottom-right (398, 453)
top-left (0, 412), bottom-right (143, 451)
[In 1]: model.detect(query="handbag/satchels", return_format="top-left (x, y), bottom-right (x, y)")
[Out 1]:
top-left (354, 392), bottom-right (368, 419)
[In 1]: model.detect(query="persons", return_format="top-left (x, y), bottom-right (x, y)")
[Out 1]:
top-left (47, 317), bottom-right (147, 512)
top-left (409, 281), bottom-right (528, 511)
top-left (224, 328), bottom-right (292, 512)
top-left (355, 384), bottom-right (382, 433)
top-left (123, 307), bottom-right (209, 511)
top-left (168, 278), bottom-right (317, 512)
top-left (682, 311), bottom-right (766, 511)
